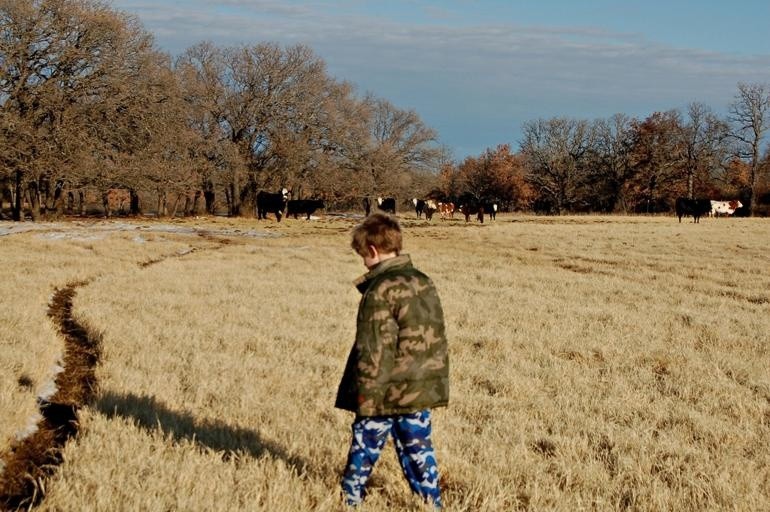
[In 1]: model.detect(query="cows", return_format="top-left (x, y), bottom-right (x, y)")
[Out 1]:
top-left (285, 197), bottom-right (325, 220)
top-left (459, 204), bottom-right (485, 224)
top-left (438, 202), bottom-right (456, 223)
top-left (485, 202), bottom-right (499, 222)
top-left (675, 197), bottom-right (712, 223)
top-left (256, 187), bottom-right (292, 223)
top-left (377, 197), bottom-right (396, 215)
top-left (708, 199), bottom-right (744, 219)
top-left (412, 196), bottom-right (437, 223)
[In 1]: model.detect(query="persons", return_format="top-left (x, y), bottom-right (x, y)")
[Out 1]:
top-left (330, 212), bottom-right (453, 512)
